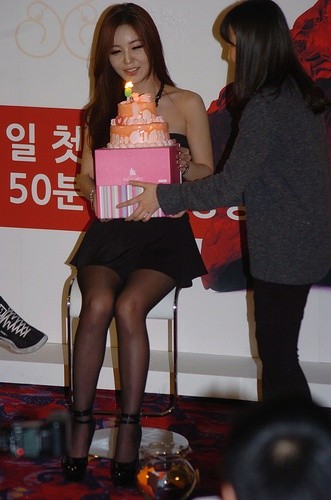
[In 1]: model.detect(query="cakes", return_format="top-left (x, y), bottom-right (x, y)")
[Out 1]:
top-left (107, 93), bottom-right (173, 148)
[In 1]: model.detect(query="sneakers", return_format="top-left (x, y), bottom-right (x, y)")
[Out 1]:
top-left (0, 295), bottom-right (48, 354)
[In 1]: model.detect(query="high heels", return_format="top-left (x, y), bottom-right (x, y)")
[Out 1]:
top-left (63, 409), bottom-right (96, 474)
top-left (111, 412), bottom-right (142, 477)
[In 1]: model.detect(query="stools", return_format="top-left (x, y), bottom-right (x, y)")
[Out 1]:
top-left (60, 227), bottom-right (191, 463)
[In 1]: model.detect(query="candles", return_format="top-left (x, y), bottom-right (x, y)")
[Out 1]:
top-left (125, 81), bottom-right (134, 100)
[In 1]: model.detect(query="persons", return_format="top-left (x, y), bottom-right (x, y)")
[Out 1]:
top-left (0, 296), bottom-right (48, 354)
top-left (116, 0), bottom-right (331, 401)
top-left (220, 401), bottom-right (331, 500)
top-left (63, 3), bottom-right (214, 490)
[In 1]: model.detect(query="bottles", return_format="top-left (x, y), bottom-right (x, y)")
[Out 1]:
top-left (136, 446), bottom-right (196, 500)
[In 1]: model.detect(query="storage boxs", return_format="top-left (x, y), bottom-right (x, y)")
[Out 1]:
top-left (94, 144), bottom-right (180, 219)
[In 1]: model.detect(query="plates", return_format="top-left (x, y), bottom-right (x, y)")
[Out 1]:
top-left (88, 426), bottom-right (189, 461)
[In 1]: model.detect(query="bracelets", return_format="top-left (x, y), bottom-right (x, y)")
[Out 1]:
top-left (182, 166), bottom-right (189, 176)
top-left (90, 189), bottom-right (94, 208)
